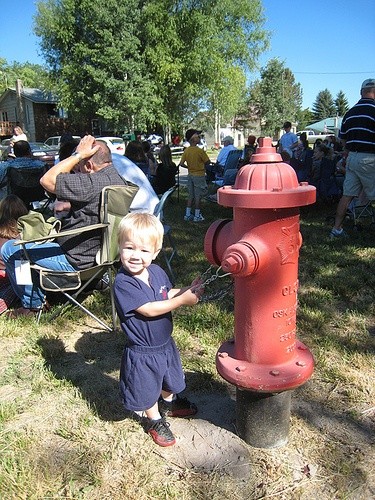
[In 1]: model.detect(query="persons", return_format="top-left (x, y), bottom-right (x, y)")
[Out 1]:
top-left (122, 140), bottom-right (155, 178)
top-left (310, 135), bottom-right (347, 197)
top-left (208, 169), bottom-right (239, 201)
top-left (54, 142), bottom-right (163, 224)
top-left (141, 141), bottom-right (158, 176)
top-left (179, 128), bottom-right (211, 222)
top-left (0, 140), bottom-right (51, 200)
top-left (205, 135), bottom-right (239, 185)
top-left (54, 135), bottom-right (80, 166)
top-left (1, 135), bottom-right (128, 320)
top-left (112, 211), bottom-right (205, 447)
top-left (173, 135), bottom-right (181, 147)
top-left (0, 194), bottom-right (29, 315)
top-left (6, 125), bottom-right (28, 161)
top-left (277, 121), bottom-right (299, 168)
top-left (240, 135), bottom-right (256, 161)
top-left (329, 78), bottom-right (374, 239)
top-left (299, 139), bottom-right (311, 162)
top-left (296, 132), bottom-right (308, 160)
top-left (150, 144), bottom-right (178, 194)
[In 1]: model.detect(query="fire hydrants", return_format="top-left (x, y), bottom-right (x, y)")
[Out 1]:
top-left (203, 137), bottom-right (317, 447)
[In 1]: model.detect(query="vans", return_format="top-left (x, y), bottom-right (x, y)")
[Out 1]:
top-left (44, 136), bottom-right (81, 154)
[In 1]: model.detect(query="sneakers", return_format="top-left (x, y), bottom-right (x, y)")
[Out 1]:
top-left (330, 228), bottom-right (349, 238)
top-left (194, 215), bottom-right (205, 221)
top-left (184, 215), bottom-right (194, 221)
top-left (147, 418), bottom-right (176, 446)
top-left (160, 394), bottom-right (198, 416)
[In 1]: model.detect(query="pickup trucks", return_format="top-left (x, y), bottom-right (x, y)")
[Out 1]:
top-left (296, 131), bottom-right (335, 144)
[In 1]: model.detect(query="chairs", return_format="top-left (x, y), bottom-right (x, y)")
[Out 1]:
top-left (1, 163), bottom-right (56, 213)
top-left (13, 181), bottom-right (140, 334)
top-left (308, 157), bottom-right (339, 201)
top-left (199, 168), bottom-right (238, 203)
top-left (153, 184), bottom-right (182, 281)
top-left (207, 150), bottom-right (243, 185)
top-left (134, 162), bottom-right (151, 181)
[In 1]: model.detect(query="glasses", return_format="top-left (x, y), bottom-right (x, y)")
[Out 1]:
top-left (193, 135), bottom-right (201, 138)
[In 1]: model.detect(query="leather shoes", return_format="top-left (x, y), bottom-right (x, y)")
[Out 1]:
top-left (6, 300), bottom-right (50, 318)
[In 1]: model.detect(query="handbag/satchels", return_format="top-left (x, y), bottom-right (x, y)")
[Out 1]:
top-left (17, 210), bottom-right (62, 245)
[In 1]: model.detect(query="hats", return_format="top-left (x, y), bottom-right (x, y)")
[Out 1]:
top-left (282, 122), bottom-right (291, 128)
top-left (221, 136), bottom-right (234, 144)
top-left (185, 129), bottom-right (202, 141)
top-left (361, 79), bottom-right (375, 88)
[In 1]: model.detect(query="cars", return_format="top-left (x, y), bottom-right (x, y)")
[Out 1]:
top-left (1, 143), bottom-right (55, 168)
top-left (95, 137), bottom-right (126, 155)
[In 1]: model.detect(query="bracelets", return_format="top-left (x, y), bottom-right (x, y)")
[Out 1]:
top-left (71, 152), bottom-right (82, 162)
